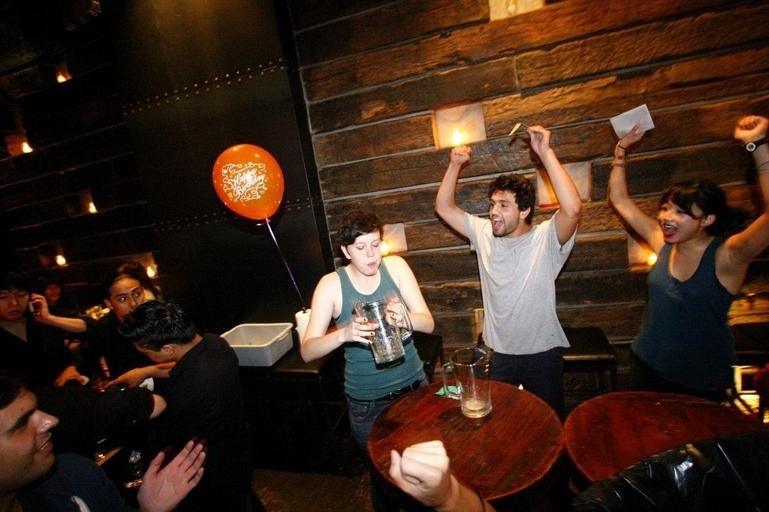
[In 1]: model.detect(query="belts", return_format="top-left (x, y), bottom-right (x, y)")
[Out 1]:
top-left (370, 380), bottom-right (419, 400)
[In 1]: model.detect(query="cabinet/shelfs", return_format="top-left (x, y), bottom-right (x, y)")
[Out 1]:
top-left (238, 343), bottom-right (343, 473)
top-left (562, 325), bottom-right (619, 398)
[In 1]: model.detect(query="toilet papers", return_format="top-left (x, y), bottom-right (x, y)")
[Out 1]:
top-left (295, 308), bottom-right (312, 345)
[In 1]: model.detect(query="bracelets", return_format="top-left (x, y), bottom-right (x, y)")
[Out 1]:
top-left (610, 161), bottom-right (628, 166)
top-left (479, 485), bottom-right (486, 511)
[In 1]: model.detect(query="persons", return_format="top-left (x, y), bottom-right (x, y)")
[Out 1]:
top-left (607, 116), bottom-right (769, 396)
top-left (0, 259), bottom-right (266, 511)
top-left (299, 210), bottom-right (437, 474)
top-left (389, 440), bottom-right (497, 510)
top-left (435, 125), bottom-right (583, 426)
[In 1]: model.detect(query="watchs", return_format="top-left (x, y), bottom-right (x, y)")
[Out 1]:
top-left (746, 136), bottom-right (768, 153)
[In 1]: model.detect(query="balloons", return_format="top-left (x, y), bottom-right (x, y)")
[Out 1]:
top-left (211, 143), bottom-right (284, 221)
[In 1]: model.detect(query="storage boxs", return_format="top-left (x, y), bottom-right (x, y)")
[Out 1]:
top-left (220, 322), bottom-right (294, 367)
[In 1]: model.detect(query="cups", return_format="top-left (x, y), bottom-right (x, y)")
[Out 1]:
top-left (442, 348), bottom-right (494, 419)
top-left (354, 297), bottom-right (414, 364)
